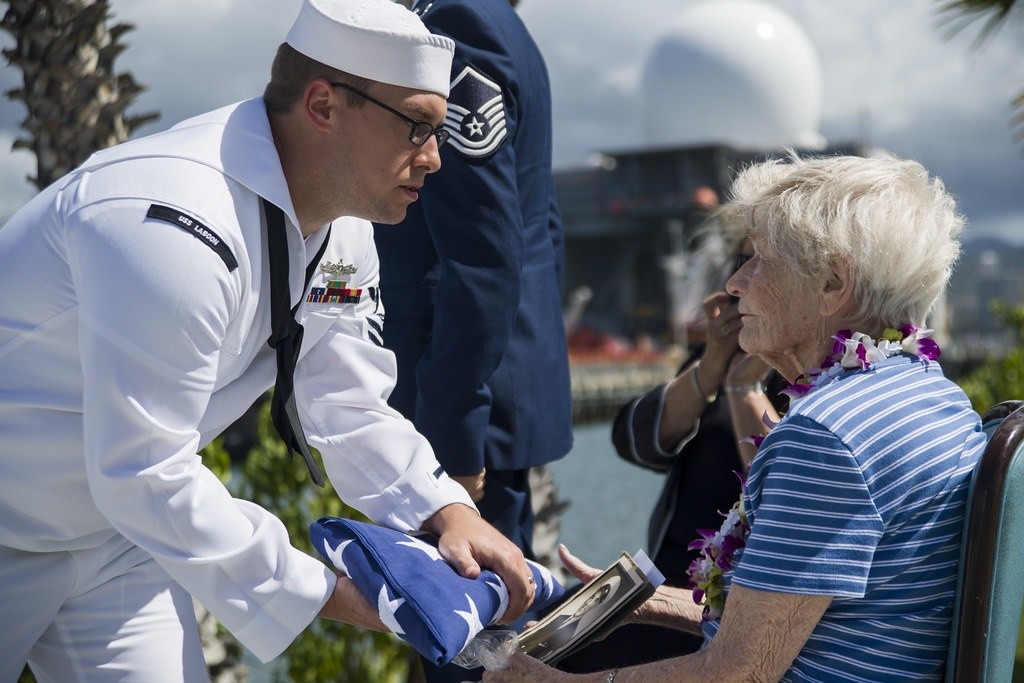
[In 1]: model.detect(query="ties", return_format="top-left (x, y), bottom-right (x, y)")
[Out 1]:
top-left (262, 198), bottom-right (332, 488)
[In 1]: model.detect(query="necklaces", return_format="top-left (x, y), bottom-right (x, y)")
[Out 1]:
top-left (685, 322), bottom-right (942, 623)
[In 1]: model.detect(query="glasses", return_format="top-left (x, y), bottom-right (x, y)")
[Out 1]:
top-left (329, 82), bottom-right (451, 151)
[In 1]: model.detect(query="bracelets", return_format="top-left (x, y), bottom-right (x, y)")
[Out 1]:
top-left (686, 358), bottom-right (718, 403)
top-left (725, 380), bottom-right (765, 392)
top-left (606, 669), bottom-right (619, 683)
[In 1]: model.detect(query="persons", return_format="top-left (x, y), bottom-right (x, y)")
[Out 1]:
top-left (375, 2), bottom-right (575, 682)
top-left (493, 146), bottom-right (990, 683)
top-left (519, 576), bottom-right (622, 662)
top-left (0, 2), bottom-right (541, 683)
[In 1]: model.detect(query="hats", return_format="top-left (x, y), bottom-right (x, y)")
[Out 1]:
top-left (286, 0), bottom-right (456, 99)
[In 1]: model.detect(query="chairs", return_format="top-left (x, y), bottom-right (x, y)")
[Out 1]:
top-left (944, 401), bottom-right (1024, 683)
